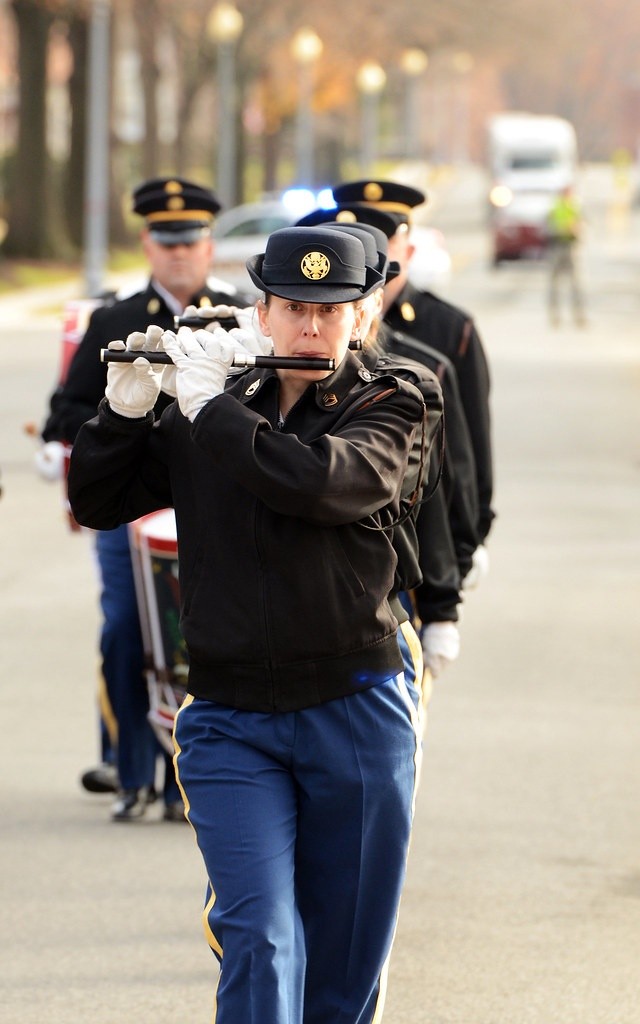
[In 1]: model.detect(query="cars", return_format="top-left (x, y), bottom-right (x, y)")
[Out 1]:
top-left (208, 200), bottom-right (452, 293)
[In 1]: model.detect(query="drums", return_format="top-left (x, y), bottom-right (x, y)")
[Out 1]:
top-left (126, 507), bottom-right (189, 758)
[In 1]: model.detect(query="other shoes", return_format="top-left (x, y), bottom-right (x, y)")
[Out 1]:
top-left (164, 802), bottom-right (188, 821)
top-left (111, 783), bottom-right (156, 819)
top-left (82, 766), bottom-right (122, 792)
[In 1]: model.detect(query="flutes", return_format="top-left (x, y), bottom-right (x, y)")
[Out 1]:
top-left (100, 314), bottom-right (362, 372)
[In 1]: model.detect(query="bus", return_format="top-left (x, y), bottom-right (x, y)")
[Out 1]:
top-left (483, 110), bottom-right (575, 263)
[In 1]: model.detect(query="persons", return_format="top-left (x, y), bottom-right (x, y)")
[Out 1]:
top-left (44, 176), bottom-right (498, 1024)
top-left (542, 185), bottom-right (592, 337)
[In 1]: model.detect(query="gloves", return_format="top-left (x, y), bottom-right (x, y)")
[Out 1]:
top-left (461, 545), bottom-right (487, 589)
top-left (37, 441), bottom-right (66, 480)
top-left (105, 304), bottom-right (275, 425)
top-left (422, 621), bottom-right (460, 680)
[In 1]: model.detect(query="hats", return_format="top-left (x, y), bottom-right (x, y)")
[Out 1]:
top-left (245, 226), bottom-right (385, 304)
top-left (133, 195), bottom-right (221, 245)
top-left (133, 177), bottom-right (205, 202)
top-left (295, 181), bottom-right (425, 286)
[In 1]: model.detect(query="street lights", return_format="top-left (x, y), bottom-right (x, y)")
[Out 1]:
top-left (204, 2), bottom-right (247, 209)
top-left (292, 27), bottom-right (323, 188)
top-left (398, 45), bottom-right (427, 184)
top-left (358, 60), bottom-right (386, 181)
